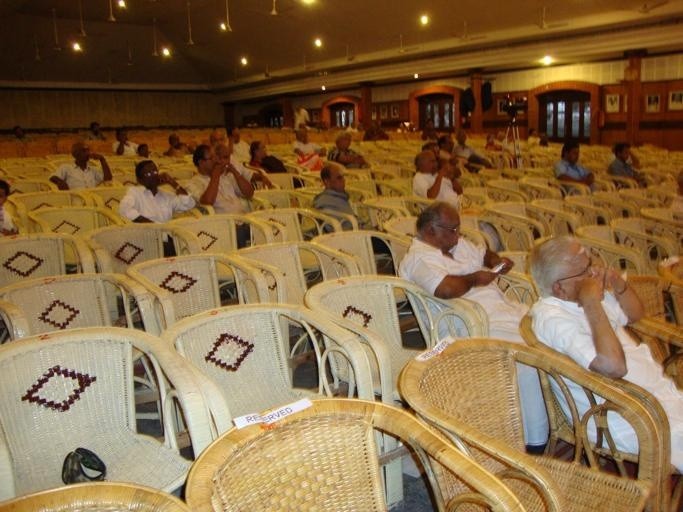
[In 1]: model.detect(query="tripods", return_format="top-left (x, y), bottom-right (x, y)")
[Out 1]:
top-left (501, 121), bottom-right (522, 166)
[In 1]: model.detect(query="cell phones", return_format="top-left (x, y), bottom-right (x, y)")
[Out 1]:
top-left (490, 262), bottom-right (507, 275)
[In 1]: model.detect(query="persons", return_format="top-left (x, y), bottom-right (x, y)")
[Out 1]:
top-left (607, 142), bottom-right (649, 193)
top-left (527, 128), bottom-right (541, 146)
top-left (527, 234), bottom-right (682, 477)
top-left (420, 119), bottom-right (438, 140)
top-left (293, 102), bottom-right (311, 135)
top-left (397, 200), bottom-right (549, 458)
top-left (551, 140), bottom-right (594, 199)
top-left (1, 121), bottom-right (513, 229)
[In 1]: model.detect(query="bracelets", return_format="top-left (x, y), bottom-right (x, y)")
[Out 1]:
top-left (615, 283), bottom-right (628, 295)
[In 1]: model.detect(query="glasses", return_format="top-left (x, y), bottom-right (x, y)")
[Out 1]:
top-left (434, 223), bottom-right (460, 233)
top-left (557, 257), bottom-right (592, 282)
